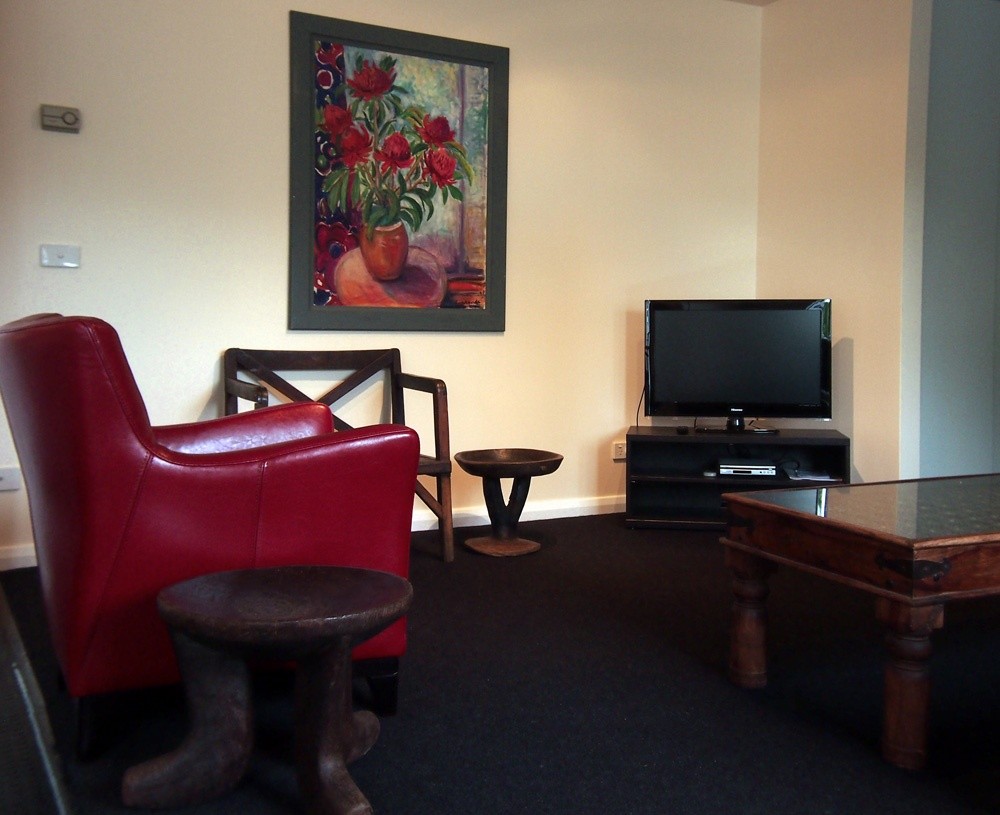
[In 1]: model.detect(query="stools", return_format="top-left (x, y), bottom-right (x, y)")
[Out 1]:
top-left (455, 447), bottom-right (564, 556)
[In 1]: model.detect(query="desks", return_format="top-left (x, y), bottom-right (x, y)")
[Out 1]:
top-left (626, 427), bottom-right (849, 529)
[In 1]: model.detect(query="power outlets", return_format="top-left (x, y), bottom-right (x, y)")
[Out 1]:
top-left (613, 442), bottom-right (626, 459)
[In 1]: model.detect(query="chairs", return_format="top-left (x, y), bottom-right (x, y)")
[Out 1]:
top-left (225, 348), bottom-right (454, 562)
top-left (723, 474), bottom-right (1000, 766)
top-left (0, 314), bottom-right (419, 762)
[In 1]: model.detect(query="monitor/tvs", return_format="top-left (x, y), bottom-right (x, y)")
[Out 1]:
top-left (643, 298), bottom-right (832, 434)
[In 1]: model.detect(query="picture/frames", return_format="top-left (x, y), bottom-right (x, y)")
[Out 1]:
top-left (287, 9), bottom-right (508, 331)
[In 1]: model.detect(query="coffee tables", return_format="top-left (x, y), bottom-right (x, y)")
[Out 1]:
top-left (123, 567), bottom-right (412, 815)
